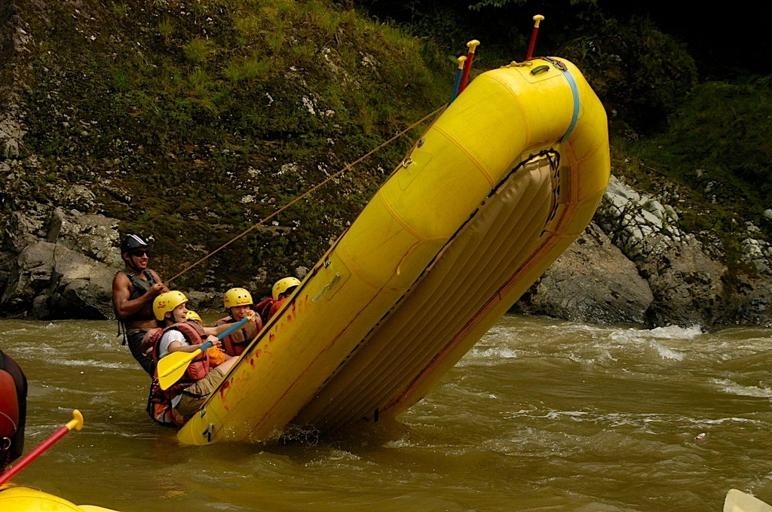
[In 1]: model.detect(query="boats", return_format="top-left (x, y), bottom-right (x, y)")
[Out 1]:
top-left (172, 57), bottom-right (611, 446)
top-left (0, 480), bottom-right (112, 511)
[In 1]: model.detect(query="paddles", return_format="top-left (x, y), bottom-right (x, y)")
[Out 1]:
top-left (156, 312), bottom-right (257, 394)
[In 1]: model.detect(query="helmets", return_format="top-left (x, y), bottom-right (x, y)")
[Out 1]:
top-left (121, 234), bottom-right (152, 250)
top-left (272, 277), bottom-right (300, 300)
top-left (153, 291), bottom-right (188, 319)
top-left (222, 287), bottom-right (254, 309)
top-left (186, 311), bottom-right (201, 322)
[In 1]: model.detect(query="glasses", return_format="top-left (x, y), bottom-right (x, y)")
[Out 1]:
top-left (131, 250), bottom-right (149, 257)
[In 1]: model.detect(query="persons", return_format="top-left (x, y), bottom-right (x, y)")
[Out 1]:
top-left (152, 291), bottom-right (255, 418)
top-left (262, 277), bottom-right (302, 327)
top-left (185, 310), bottom-right (203, 327)
top-left (213, 287), bottom-right (262, 356)
top-left (112, 234), bottom-right (170, 379)
